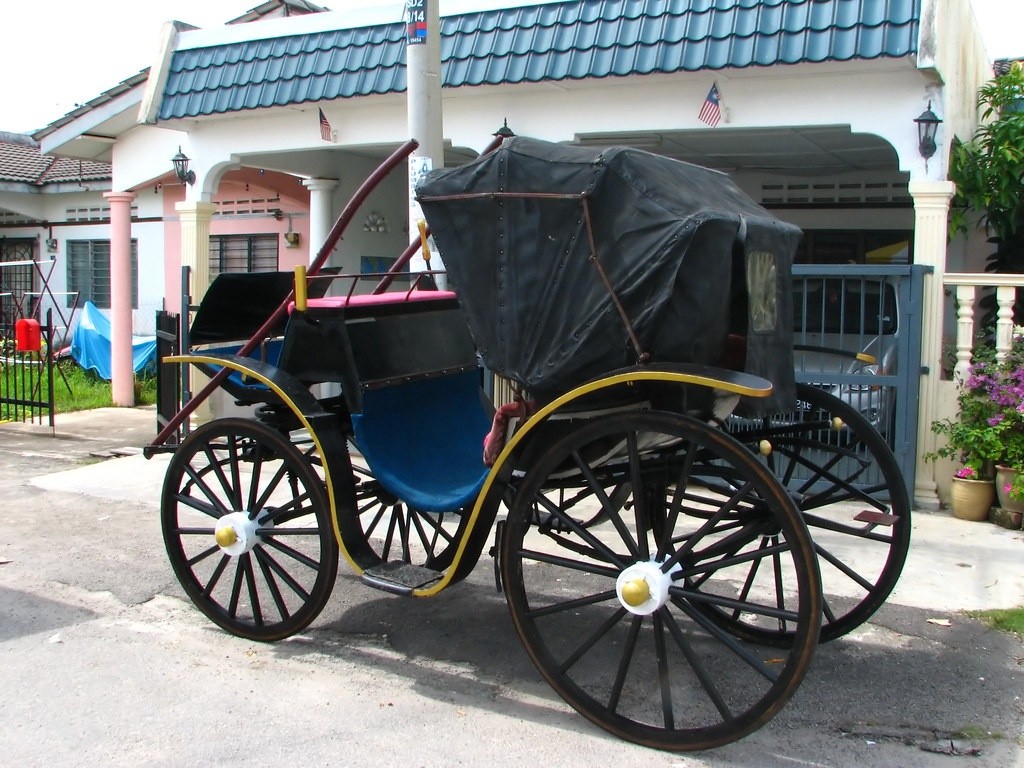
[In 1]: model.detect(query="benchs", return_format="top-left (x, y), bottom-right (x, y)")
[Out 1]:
top-left (263, 253), bottom-right (460, 387)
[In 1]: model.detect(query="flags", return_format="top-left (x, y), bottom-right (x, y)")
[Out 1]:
top-left (319, 108), bottom-right (332, 141)
top-left (698, 80), bottom-right (722, 128)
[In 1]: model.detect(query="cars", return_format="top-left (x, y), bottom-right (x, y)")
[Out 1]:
top-left (790, 275), bottom-right (901, 451)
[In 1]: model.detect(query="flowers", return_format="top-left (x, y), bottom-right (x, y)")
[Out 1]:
top-left (920, 343), bottom-right (1024, 501)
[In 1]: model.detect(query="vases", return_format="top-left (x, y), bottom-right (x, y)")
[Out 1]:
top-left (992, 457), bottom-right (1024, 512)
top-left (948, 473), bottom-right (990, 518)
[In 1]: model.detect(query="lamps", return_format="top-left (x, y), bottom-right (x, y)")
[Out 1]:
top-left (170, 141), bottom-right (199, 190)
top-left (282, 230), bottom-right (301, 248)
top-left (914, 97), bottom-right (943, 162)
